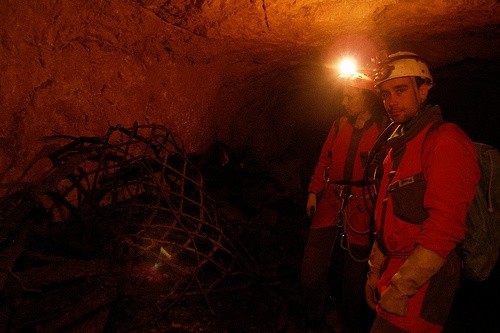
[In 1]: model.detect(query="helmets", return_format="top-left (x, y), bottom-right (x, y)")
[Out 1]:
top-left (338, 66), bottom-right (374, 90)
top-left (373, 49), bottom-right (435, 91)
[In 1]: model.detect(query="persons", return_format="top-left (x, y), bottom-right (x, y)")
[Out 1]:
top-left (364, 51), bottom-right (480, 333)
top-left (297, 68), bottom-right (388, 333)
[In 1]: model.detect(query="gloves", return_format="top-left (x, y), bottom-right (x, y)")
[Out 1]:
top-left (377, 245), bottom-right (447, 317)
top-left (365, 239), bottom-right (387, 310)
top-left (306, 192), bottom-right (317, 216)
